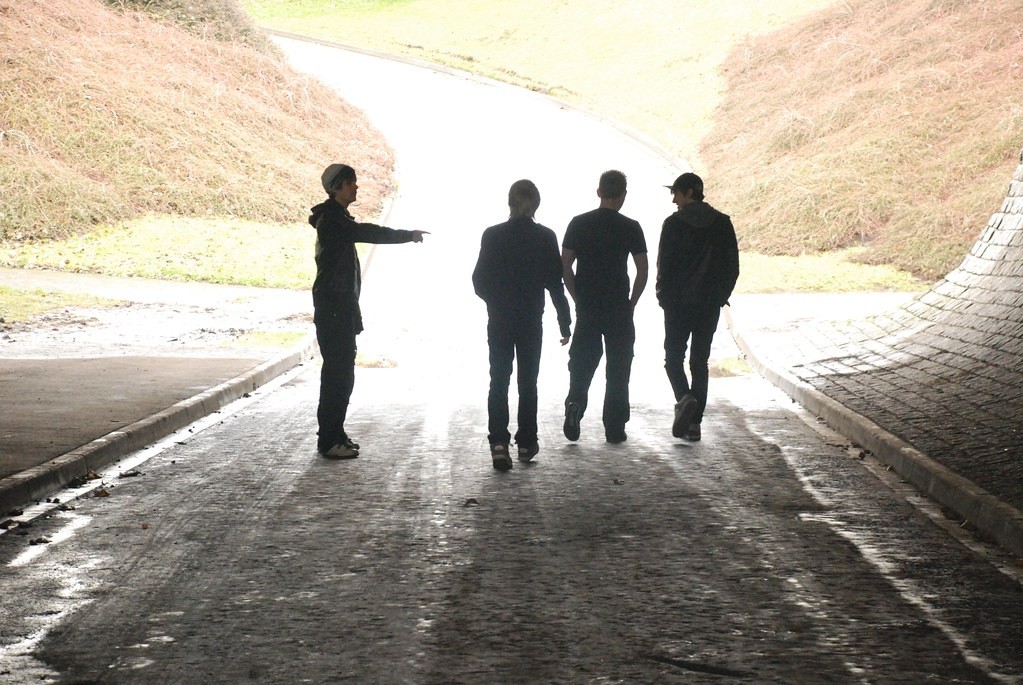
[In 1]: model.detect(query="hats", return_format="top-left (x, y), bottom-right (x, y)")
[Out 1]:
top-left (664, 172), bottom-right (703, 194)
top-left (321, 163), bottom-right (356, 194)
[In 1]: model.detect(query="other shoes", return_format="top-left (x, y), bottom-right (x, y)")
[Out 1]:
top-left (607, 432), bottom-right (627, 443)
top-left (564, 401), bottom-right (581, 442)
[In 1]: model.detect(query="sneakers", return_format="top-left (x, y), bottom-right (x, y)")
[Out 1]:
top-left (518, 442), bottom-right (539, 463)
top-left (489, 440), bottom-right (513, 468)
top-left (322, 443), bottom-right (359, 458)
top-left (315, 438), bottom-right (361, 452)
top-left (684, 421), bottom-right (702, 440)
top-left (671, 393), bottom-right (698, 438)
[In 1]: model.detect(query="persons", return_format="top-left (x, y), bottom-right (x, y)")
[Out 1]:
top-left (560, 169), bottom-right (650, 442)
top-left (654, 171), bottom-right (739, 442)
top-left (472, 177), bottom-right (573, 472)
top-left (309, 163), bottom-right (432, 464)
